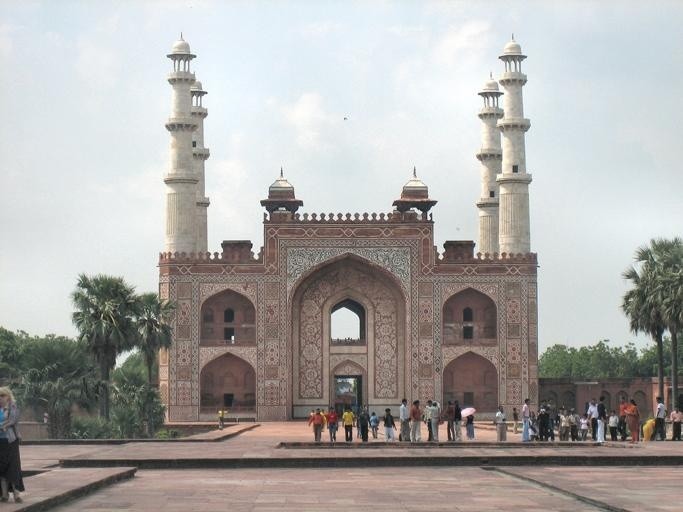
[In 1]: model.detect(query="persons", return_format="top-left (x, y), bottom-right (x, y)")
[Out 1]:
top-left (309, 404), bottom-right (379, 443)
top-left (0, 387), bottom-right (24, 503)
top-left (400, 398), bottom-right (476, 442)
top-left (383, 408), bottom-right (397, 442)
top-left (493, 406), bottom-right (508, 441)
top-left (219, 409), bottom-right (228, 429)
top-left (512, 397), bottom-right (682, 441)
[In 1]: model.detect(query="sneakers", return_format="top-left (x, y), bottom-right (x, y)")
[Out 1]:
top-left (14, 496), bottom-right (23, 503)
top-left (0, 495), bottom-right (10, 502)
